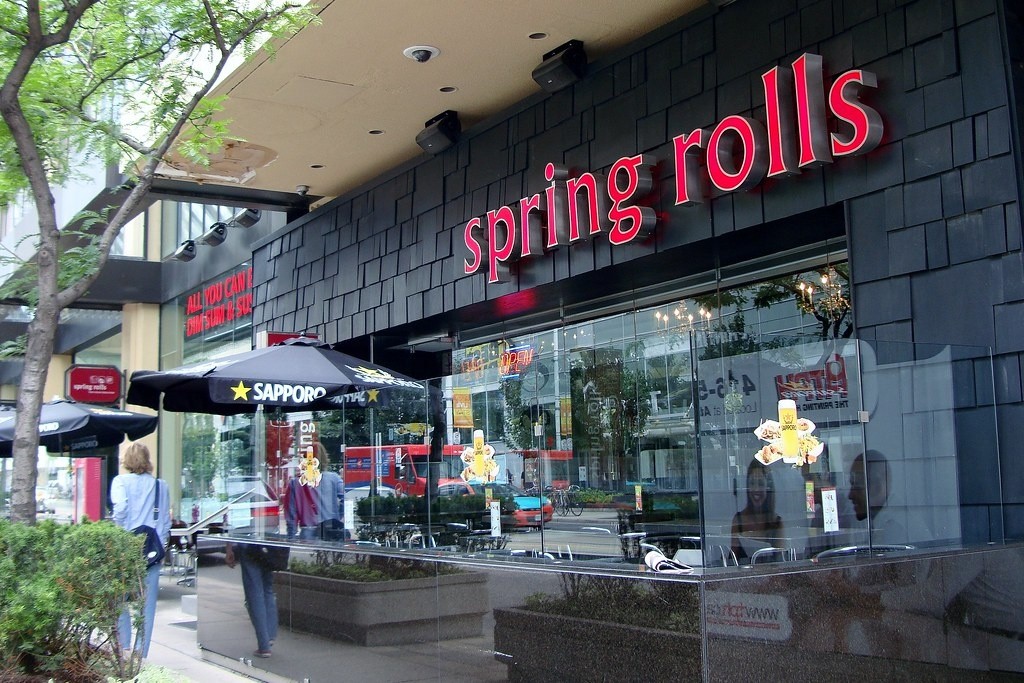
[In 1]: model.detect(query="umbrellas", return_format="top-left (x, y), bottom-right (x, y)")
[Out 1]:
top-left (0, 395), bottom-right (159, 458)
top-left (125, 331), bottom-right (445, 422)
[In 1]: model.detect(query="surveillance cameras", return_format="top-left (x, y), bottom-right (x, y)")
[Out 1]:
top-left (412, 49), bottom-right (431, 63)
top-left (297, 185), bottom-right (309, 196)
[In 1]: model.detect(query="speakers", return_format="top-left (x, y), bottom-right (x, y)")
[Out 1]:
top-left (531, 44), bottom-right (588, 92)
top-left (415, 117), bottom-right (461, 155)
top-left (203, 224), bottom-right (228, 246)
top-left (175, 241), bottom-right (197, 262)
top-left (235, 208), bottom-right (261, 227)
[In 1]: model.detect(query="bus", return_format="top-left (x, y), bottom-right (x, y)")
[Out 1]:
top-left (344, 444), bottom-right (470, 498)
top-left (492, 449), bottom-right (573, 492)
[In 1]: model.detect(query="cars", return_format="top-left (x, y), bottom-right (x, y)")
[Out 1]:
top-left (437, 480), bottom-right (556, 527)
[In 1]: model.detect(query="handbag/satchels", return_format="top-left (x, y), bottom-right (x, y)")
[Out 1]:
top-left (317, 518), bottom-right (345, 541)
top-left (127, 525), bottom-right (166, 571)
top-left (256, 544), bottom-right (290, 572)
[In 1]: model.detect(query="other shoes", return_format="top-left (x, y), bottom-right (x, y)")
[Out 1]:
top-left (120, 647), bottom-right (132, 663)
top-left (254, 650), bottom-right (271, 658)
top-left (269, 639), bottom-right (275, 647)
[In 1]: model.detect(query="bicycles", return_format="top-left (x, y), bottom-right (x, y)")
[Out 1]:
top-left (545, 485), bottom-right (589, 515)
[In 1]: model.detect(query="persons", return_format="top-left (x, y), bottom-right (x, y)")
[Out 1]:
top-left (110, 443), bottom-right (171, 671)
top-left (727, 457), bottom-right (787, 565)
top-left (225, 543), bottom-right (281, 658)
top-left (283, 441), bottom-right (346, 540)
top-left (847, 449), bottom-right (933, 547)
